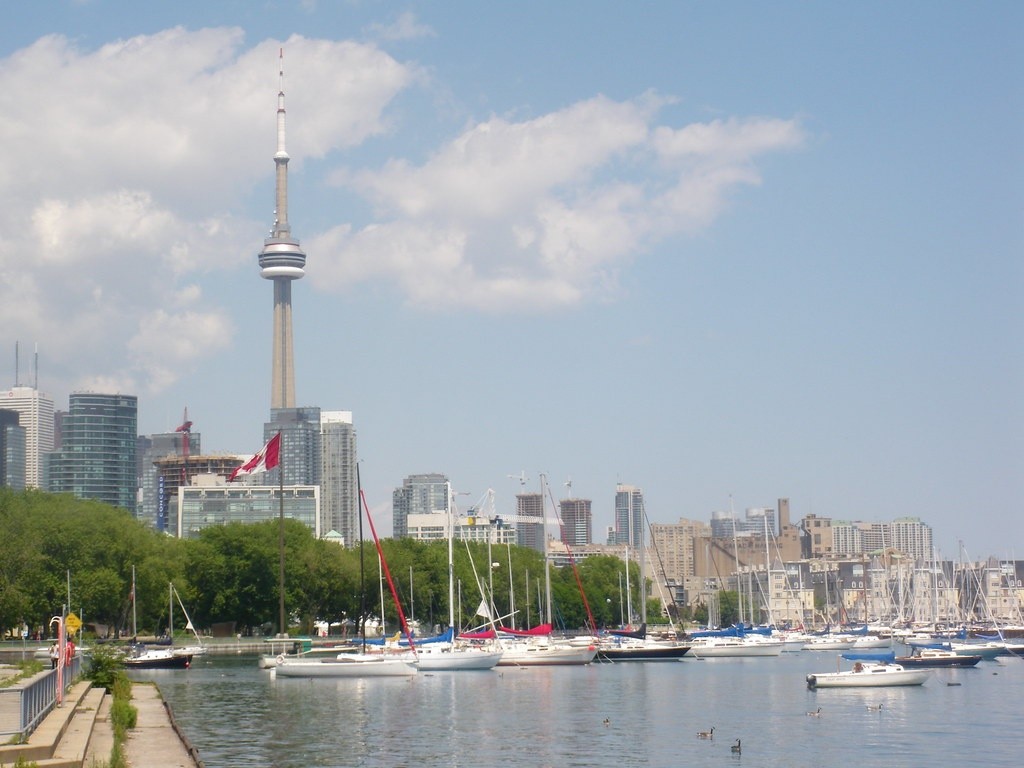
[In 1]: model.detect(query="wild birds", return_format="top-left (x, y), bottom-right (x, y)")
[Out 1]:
top-left (405, 675), bottom-right (415, 683)
top-left (730, 737), bottom-right (741, 751)
top-left (498, 671), bottom-right (505, 678)
top-left (805, 705), bottom-right (824, 719)
top-left (602, 715), bottom-right (611, 726)
top-left (696, 726), bottom-right (717, 737)
top-left (864, 702), bottom-right (884, 711)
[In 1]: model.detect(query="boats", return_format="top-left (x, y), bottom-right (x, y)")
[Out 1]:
top-left (805, 661), bottom-right (932, 686)
top-left (894, 642), bottom-right (984, 664)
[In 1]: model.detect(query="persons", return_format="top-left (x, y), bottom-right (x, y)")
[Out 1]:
top-left (65, 638), bottom-right (77, 668)
top-left (50, 641), bottom-right (60, 670)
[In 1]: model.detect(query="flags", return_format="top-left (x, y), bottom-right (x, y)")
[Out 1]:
top-left (228, 433), bottom-right (280, 482)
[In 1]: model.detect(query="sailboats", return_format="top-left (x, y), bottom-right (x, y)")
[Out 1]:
top-left (839, 522), bottom-right (888, 661)
top-left (909, 542), bottom-right (1024, 656)
top-left (32, 564), bottom-right (204, 669)
top-left (258, 429), bottom-right (1023, 677)
